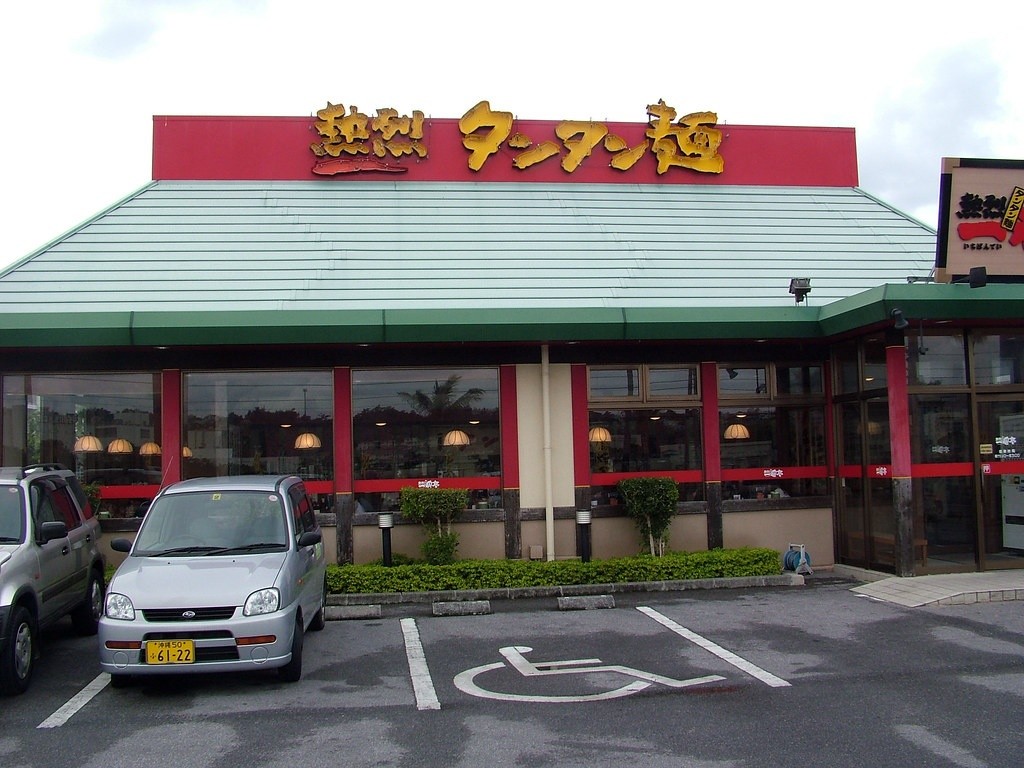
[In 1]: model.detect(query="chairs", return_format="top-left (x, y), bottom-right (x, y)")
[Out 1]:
top-left (188, 514), bottom-right (217, 543)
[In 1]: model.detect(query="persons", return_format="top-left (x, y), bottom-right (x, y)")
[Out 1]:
top-left (480, 459), bottom-right (494, 473)
top-left (229, 419), bottom-right (262, 475)
top-left (359, 441), bottom-right (384, 512)
top-left (756, 491), bottom-right (764, 499)
top-left (312, 423), bottom-right (333, 513)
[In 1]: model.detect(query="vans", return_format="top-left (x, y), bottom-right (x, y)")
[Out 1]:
top-left (97, 474), bottom-right (328, 684)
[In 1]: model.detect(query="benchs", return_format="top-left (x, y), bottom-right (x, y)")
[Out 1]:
top-left (848, 529), bottom-right (928, 569)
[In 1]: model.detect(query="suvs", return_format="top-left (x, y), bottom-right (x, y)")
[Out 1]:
top-left (0, 465), bottom-right (106, 695)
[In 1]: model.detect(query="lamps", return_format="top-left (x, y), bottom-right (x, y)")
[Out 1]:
top-left (724, 424), bottom-right (750, 439)
top-left (442, 429), bottom-right (471, 446)
top-left (183, 445), bottom-right (193, 458)
top-left (106, 438), bottom-right (135, 455)
top-left (73, 431), bottom-right (103, 454)
top-left (294, 432), bottom-right (322, 450)
top-left (589, 426), bottom-right (612, 442)
top-left (139, 441), bottom-right (162, 456)
top-left (726, 368), bottom-right (738, 379)
